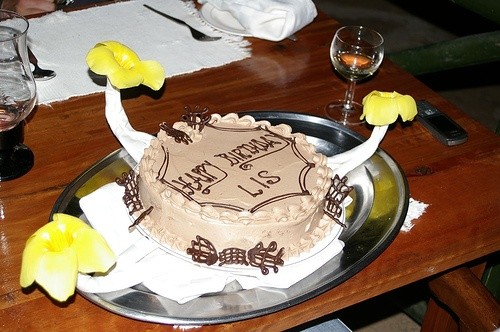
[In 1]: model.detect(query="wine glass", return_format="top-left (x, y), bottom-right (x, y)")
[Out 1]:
top-left (0, 9), bottom-right (37, 181)
top-left (326, 25), bottom-right (385, 126)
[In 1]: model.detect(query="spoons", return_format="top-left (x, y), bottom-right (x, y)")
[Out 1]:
top-left (26, 46), bottom-right (56, 82)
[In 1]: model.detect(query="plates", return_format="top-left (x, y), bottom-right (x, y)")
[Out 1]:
top-left (47, 111), bottom-right (409, 325)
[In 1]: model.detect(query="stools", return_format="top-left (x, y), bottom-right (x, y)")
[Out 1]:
top-left (420, 265), bottom-right (499, 330)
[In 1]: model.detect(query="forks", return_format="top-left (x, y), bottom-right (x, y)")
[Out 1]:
top-left (144, 3), bottom-right (222, 42)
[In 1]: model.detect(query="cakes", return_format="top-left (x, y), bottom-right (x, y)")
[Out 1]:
top-left (20, 41), bottom-right (419, 302)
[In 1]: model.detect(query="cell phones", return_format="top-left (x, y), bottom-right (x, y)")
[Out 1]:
top-left (413, 98), bottom-right (470, 146)
